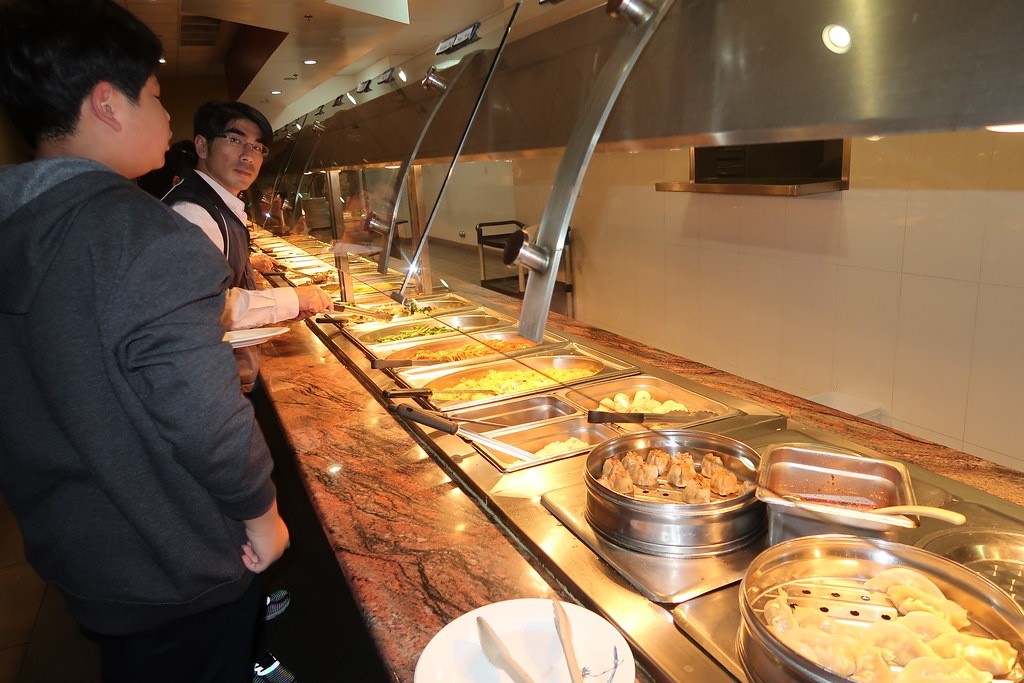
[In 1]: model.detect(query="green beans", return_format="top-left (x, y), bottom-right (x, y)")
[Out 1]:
top-left (376, 325), bottom-right (458, 344)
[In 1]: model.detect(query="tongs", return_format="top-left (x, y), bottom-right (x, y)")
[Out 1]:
top-left (476, 596), bottom-right (583, 683)
top-left (586, 409), bottom-right (721, 424)
top-left (381, 388), bottom-right (535, 462)
top-left (327, 302), bottom-right (394, 322)
top-left (262, 264), bottom-right (310, 278)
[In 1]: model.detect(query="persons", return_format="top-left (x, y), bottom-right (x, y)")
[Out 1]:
top-left (0, 0), bottom-right (290, 683)
top-left (155, 100), bottom-right (335, 395)
top-left (255, 169), bottom-right (404, 261)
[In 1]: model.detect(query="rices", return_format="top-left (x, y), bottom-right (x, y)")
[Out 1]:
top-left (431, 366), bottom-right (593, 401)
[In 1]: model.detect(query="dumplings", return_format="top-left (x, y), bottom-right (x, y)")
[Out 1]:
top-left (761, 566), bottom-right (1019, 683)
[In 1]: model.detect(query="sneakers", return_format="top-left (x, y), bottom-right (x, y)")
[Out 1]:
top-left (253, 650), bottom-right (297, 683)
top-left (264, 589), bottom-right (290, 620)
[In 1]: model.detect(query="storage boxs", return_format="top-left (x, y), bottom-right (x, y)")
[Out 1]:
top-left (755, 443), bottom-right (921, 546)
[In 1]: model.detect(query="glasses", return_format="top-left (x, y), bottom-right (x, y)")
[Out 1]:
top-left (207, 133), bottom-right (269, 156)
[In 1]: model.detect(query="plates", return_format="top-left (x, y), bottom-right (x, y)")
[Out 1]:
top-left (412, 598), bottom-right (636, 683)
top-left (223, 327), bottom-right (291, 349)
